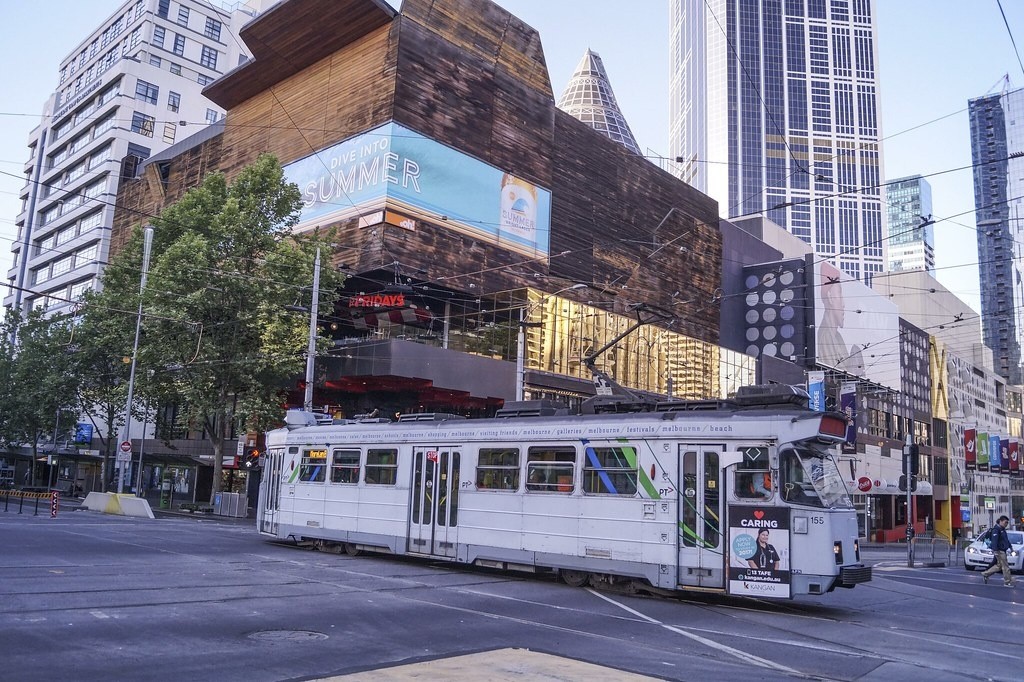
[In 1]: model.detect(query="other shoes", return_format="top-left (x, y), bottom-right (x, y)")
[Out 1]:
top-left (1003, 582), bottom-right (1015, 588)
top-left (981, 572), bottom-right (989, 584)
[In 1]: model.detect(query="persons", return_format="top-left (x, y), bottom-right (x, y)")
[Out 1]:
top-left (745, 528), bottom-right (780, 570)
top-left (817, 261), bottom-right (867, 378)
top-left (906, 523), bottom-right (916, 546)
top-left (980, 515), bottom-right (1017, 588)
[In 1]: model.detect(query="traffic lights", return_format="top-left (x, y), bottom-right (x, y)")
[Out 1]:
top-left (242, 446), bottom-right (260, 471)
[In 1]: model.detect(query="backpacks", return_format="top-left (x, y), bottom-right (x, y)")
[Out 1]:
top-left (984, 528), bottom-right (1002, 549)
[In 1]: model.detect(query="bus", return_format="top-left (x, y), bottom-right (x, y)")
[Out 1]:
top-left (256, 382), bottom-right (874, 604)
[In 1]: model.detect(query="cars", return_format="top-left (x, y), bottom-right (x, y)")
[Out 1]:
top-left (963, 530), bottom-right (1024, 574)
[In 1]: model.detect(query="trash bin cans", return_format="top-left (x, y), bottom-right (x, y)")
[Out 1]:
top-left (213, 491), bottom-right (246, 517)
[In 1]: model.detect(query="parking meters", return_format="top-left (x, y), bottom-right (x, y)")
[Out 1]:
top-left (952, 528), bottom-right (962, 565)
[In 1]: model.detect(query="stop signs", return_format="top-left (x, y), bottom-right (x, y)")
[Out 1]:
top-left (121, 442), bottom-right (131, 451)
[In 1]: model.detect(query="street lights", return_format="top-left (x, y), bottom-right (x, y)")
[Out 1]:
top-left (515, 283), bottom-right (589, 398)
